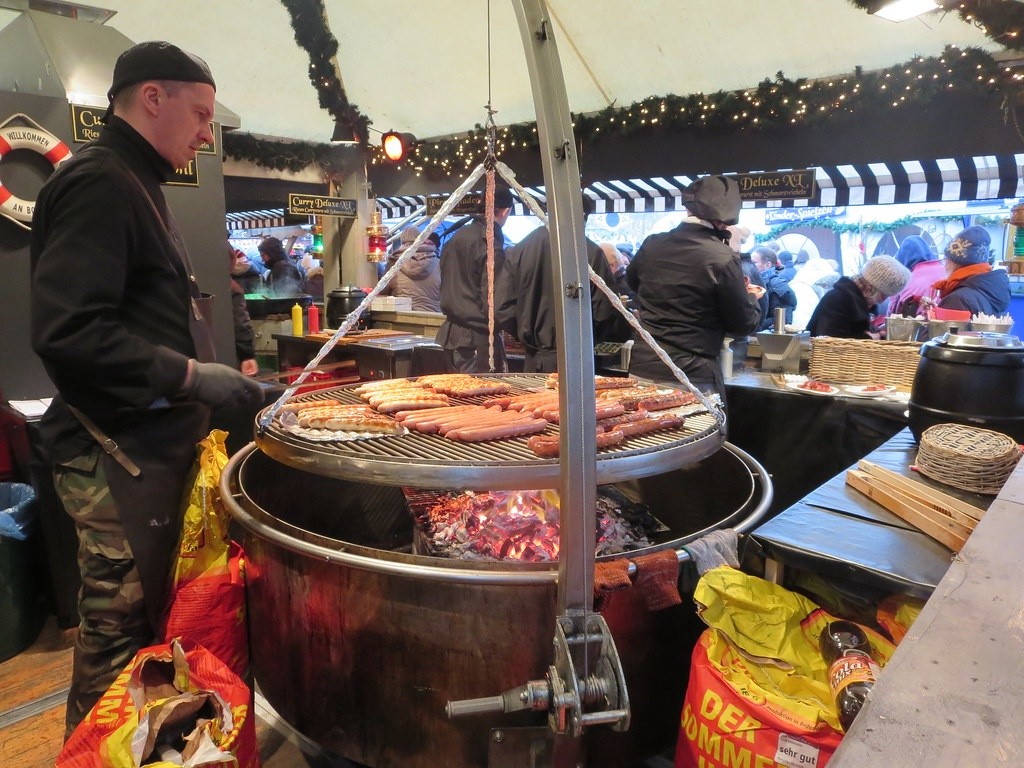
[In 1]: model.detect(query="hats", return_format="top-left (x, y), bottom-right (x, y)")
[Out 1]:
top-left (258, 237), bottom-right (283, 256)
top-left (598, 243), bottom-right (617, 264)
top-left (898, 235), bottom-right (932, 268)
top-left (944, 226), bottom-right (991, 264)
top-left (401, 226), bottom-right (420, 244)
top-left (301, 253), bottom-right (320, 269)
top-left (108, 41), bottom-right (216, 103)
top-left (617, 242), bottom-right (634, 253)
top-left (861, 255), bottom-right (911, 296)
top-left (481, 184), bottom-right (513, 207)
top-left (681, 175), bottom-right (741, 225)
top-left (727, 226), bottom-right (751, 252)
top-left (581, 192), bottom-right (593, 214)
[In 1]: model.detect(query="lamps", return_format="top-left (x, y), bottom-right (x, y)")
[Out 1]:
top-left (381, 132), bottom-right (426, 164)
top-left (367, 212), bottom-right (388, 263)
top-left (311, 216), bottom-right (323, 259)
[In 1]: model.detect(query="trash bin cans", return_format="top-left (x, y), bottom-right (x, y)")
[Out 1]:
top-left (0, 482), bottom-right (49, 662)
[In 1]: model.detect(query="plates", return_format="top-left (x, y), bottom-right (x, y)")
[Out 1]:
top-left (748, 283), bottom-right (766, 294)
top-left (845, 385), bottom-right (897, 396)
top-left (787, 383), bottom-right (839, 396)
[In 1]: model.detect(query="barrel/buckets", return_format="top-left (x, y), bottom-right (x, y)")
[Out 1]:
top-left (885, 317), bottom-right (925, 342)
top-left (308, 299), bottom-right (318, 334)
top-left (969, 323), bottom-right (1013, 334)
top-left (927, 319), bottom-right (969, 341)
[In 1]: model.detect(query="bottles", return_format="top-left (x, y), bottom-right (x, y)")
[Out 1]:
top-left (819, 621), bottom-right (879, 732)
top-left (292, 302), bottom-right (303, 337)
top-left (719, 338), bottom-right (734, 379)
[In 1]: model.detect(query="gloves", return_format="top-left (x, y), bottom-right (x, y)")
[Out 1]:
top-left (187, 359), bottom-right (265, 411)
top-left (632, 549), bottom-right (682, 610)
top-left (680, 528), bottom-right (740, 576)
top-left (592, 558), bottom-right (631, 611)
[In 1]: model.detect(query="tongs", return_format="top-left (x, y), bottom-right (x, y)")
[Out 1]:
top-left (846, 459), bottom-right (987, 554)
top-left (770, 373), bottom-right (785, 388)
top-left (249, 360), bottom-right (361, 393)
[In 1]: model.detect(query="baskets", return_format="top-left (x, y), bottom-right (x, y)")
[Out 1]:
top-left (809, 336), bottom-right (924, 386)
top-left (915, 423), bottom-right (1023, 494)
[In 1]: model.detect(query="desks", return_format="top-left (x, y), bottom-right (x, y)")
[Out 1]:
top-left (604, 366), bottom-right (911, 519)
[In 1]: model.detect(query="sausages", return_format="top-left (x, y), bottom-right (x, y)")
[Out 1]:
top-left (274, 401), bottom-right (404, 434)
top-left (354, 373), bottom-right (561, 457)
top-left (594, 377), bottom-right (696, 448)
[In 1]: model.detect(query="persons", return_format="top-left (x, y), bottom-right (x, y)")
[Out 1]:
top-left (378, 224), bottom-right (444, 312)
top-left (916, 223), bottom-right (1011, 342)
top-left (22, 40), bottom-right (267, 743)
top-left (722, 223), bottom-right (839, 334)
top-left (599, 242), bottom-right (639, 309)
top-left (804, 254), bottom-right (911, 340)
top-left (232, 248), bottom-right (261, 295)
top-left (435, 182), bottom-right (513, 372)
top-left (303, 258), bottom-right (324, 301)
top-left (885, 233), bottom-right (950, 316)
top-left (624, 173), bottom-right (763, 415)
top-left (257, 236), bottom-right (302, 292)
top-left (226, 239), bottom-right (259, 375)
top-left (492, 191), bottom-right (634, 375)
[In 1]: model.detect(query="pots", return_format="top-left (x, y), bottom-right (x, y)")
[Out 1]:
top-left (325, 284), bottom-right (370, 331)
top-left (243, 294), bottom-right (313, 320)
top-left (909, 326), bottom-right (1024, 446)
